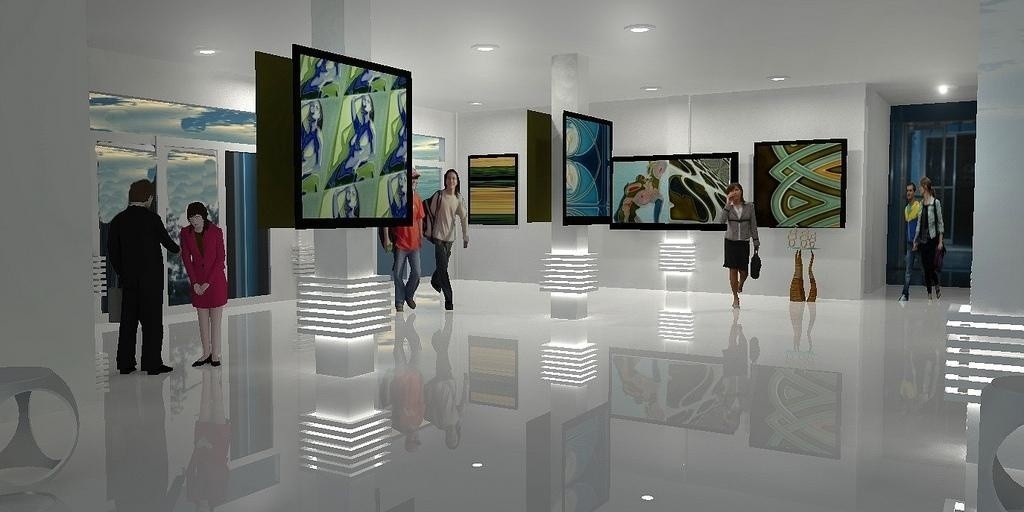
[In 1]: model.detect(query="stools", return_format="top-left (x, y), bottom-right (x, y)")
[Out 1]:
top-left (0, 366), bottom-right (80, 497)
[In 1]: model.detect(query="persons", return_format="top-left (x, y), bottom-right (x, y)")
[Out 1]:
top-left (180, 201), bottom-right (227, 367)
top-left (186, 367), bottom-right (231, 512)
top-left (383, 168), bottom-right (426, 313)
top-left (425, 311), bottom-right (469, 452)
top-left (721, 307), bottom-right (750, 431)
top-left (899, 305), bottom-right (919, 414)
top-left (720, 183), bottom-right (759, 307)
top-left (391, 313), bottom-right (424, 459)
top-left (922, 306), bottom-right (938, 408)
top-left (425, 168), bottom-right (471, 311)
top-left (897, 183), bottom-right (926, 306)
top-left (911, 175), bottom-right (946, 305)
top-left (302, 55), bottom-right (409, 220)
top-left (106, 178), bottom-right (180, 375)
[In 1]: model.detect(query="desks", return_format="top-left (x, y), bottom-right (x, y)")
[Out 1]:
top-left (790, 248), bottom-right (821, 303)
